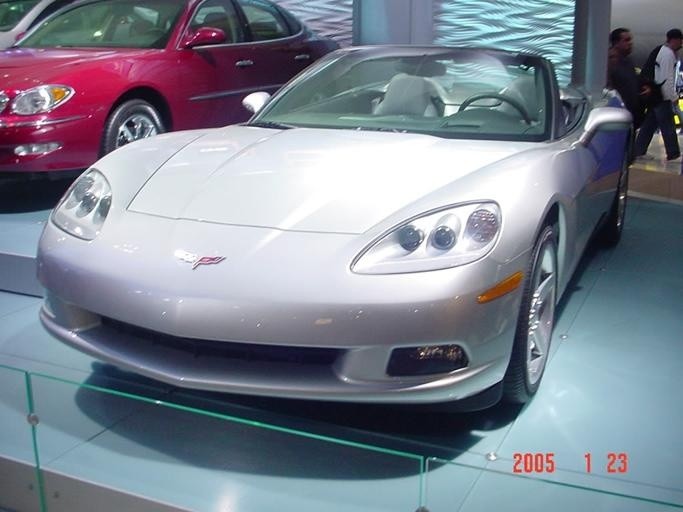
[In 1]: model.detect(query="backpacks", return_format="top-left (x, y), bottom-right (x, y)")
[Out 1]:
top-left (640, 44), bottom-right (675, 89)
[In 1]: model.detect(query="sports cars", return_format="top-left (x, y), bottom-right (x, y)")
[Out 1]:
top-left (36, 42), bottom-right (636, 413)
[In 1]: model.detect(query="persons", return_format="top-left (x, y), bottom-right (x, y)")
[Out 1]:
top-left (608, 27), bottom-right (648, 131)
top-left (633, 27), bottom-right (682, 165)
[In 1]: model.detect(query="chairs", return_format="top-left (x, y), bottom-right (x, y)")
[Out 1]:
top-left (126, 13), bottom-right (284, 43)
top-left (371, 71), bottom-right (570, 128)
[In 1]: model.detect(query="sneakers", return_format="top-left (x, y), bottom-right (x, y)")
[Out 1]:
top-left (634, 153), bottom-right (683, 162)
